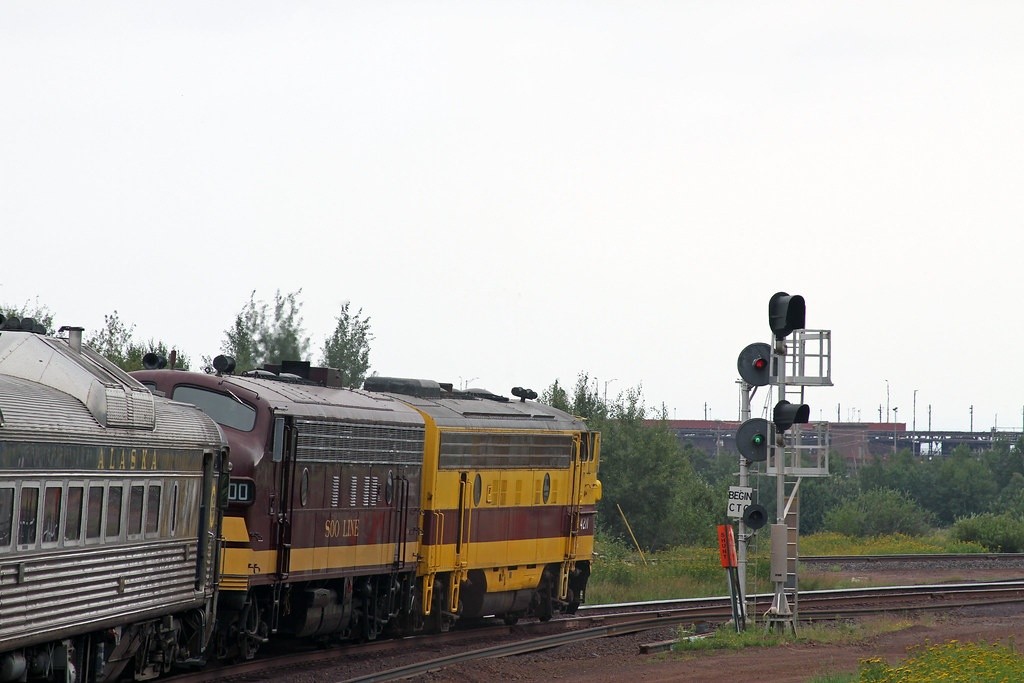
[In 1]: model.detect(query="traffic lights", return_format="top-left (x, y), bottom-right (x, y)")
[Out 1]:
top-left (773, 399), bottom-right (810, 435)
top-left (736, 417), bottom-right (776, 461)
top-left (737, 343), bottom-right (778, 387)
top-left (743, 504), bottom-right (769, 529)
top-left (769, 292), bottom-right (805, 341)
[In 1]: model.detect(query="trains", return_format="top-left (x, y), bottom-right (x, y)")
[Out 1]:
top-left (0, 327), bottom-right (604, 683)
